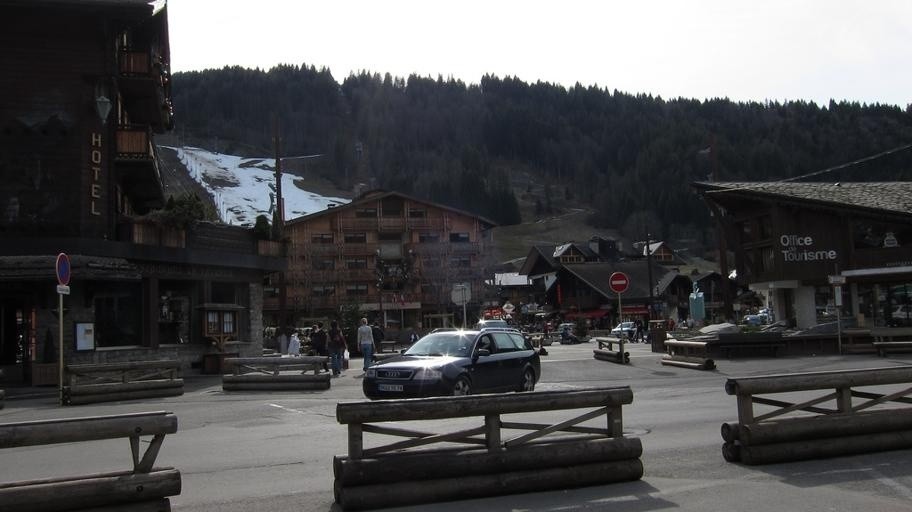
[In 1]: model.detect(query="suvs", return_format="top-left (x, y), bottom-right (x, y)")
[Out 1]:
top-left (363, 326), bottom-right (541, 399)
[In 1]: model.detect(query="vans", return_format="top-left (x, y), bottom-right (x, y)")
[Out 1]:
top-left (557, 323), bottom-right (576, 339)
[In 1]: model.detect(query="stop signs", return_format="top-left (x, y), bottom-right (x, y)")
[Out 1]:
top-left (609, 272), bottom-right (629, 293)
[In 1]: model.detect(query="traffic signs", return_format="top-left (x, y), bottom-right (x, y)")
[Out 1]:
top-left (829, 275), bottom-right (846, 283)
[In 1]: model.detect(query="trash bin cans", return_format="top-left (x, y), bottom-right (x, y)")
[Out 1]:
top-left (649, 320), bottom-right (667, 352)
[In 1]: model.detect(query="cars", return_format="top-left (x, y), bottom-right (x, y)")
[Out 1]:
top-left (611, 322), bottom-right (637, 338)
top-left (474, 320), bottom-right (507, 330)
top-left (888, 305), bottom-right (912, 319)
top-left (742, 307), bottom-right (770, 324)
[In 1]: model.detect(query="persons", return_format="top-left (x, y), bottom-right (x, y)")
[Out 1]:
top-left (409, 330), bottom-right (420, 344)
top-left (533, 319), bottom-right (581, 345)
top-left (275, 320), bottom-right (349, 378)
top-left (357, 318), bottom-right (375, 373)
top-left (370, 321), bottom-right (382, 357)
top-left (629, 314), bottom-right (705, 342)
